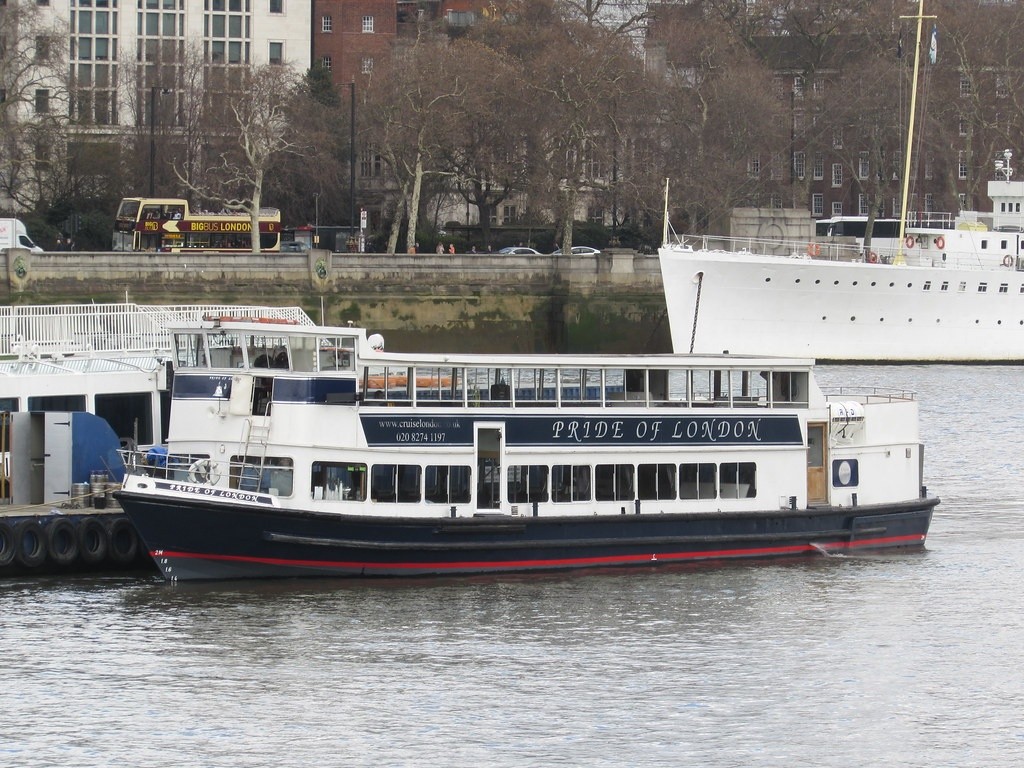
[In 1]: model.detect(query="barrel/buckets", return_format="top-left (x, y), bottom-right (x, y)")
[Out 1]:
top-left (71, 471), bottom-right (122, 509)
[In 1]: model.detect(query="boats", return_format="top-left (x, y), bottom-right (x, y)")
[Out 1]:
top-left (112, 311), bottom-right (943, 588)
top-left (657, 1), bottom-right (1024, 367)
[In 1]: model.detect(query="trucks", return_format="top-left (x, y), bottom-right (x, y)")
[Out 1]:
top-left (0, 219), bottom-right (44, 253)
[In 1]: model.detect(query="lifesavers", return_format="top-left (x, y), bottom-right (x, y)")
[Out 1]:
top-left (16, 519), bottom-right (47, 565)
top-left (188, 458), bottom-right (222, 486)
top-left (868, 252), bottom-right (878, 263)
top-left (1003, 255), bottom-right (1014, 267)
top-left (0, 519), bottom-right (17, 566)
top-left (937, 237), bottom-right (945, 250)
top-left (808, 244), bottom-right (821, 257)
top-left (47, 518), bottom-right (77, 562)
top-left (109, 518), bottom-right (138, 562)
top-left (77, 518), bottom-right (109, 564)
top-left (906, 236), bottom-right (914, 249)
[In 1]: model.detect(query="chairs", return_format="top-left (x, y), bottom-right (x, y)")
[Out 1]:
top-left (366, 386), bottom-right (624, 400)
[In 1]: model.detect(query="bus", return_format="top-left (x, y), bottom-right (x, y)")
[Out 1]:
top-left (826, 216), bottom-right (906, 247)
top-left (814, 219), bottom-right (831, 238)
top-left (111, 197), bottom-right (282, 253)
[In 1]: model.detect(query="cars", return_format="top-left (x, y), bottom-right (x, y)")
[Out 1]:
top-left (497, 247), bottom-right (542, 255)
top-left (551, 246), bottom-right (600, 256)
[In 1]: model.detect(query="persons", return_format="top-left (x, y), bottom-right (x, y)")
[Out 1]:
top-left (141, 206), bottom-right (238, 219)
top-left (483, 243), bottom-right (498, 253)
top-left (212, 236), bottom-right (251, 248)
top-left (435, 241), bottom-right (445, 254)
top-left (448, 243), bottom-right (456, 254)
top-left (416, 241), bottom-right (420, 252)
top-left (469, 245), bottom-right (478, 254)
